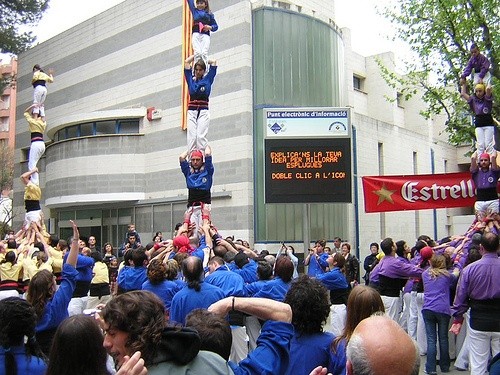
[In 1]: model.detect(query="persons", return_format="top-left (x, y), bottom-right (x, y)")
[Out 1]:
top-left (46, 315), bottom-right (112, 375)
top-left (309, 314), bottom-right (421, 375)
top-left (0, 297), bottom-right (48, 375)
top-left (423, 255), bottom-right (460, 375)
top-left (362, 151), bottom-right (500, 370)
top-left (185, 296), bottom-right (294, 375)
top-left (114, 351), bottom-right (147, 375)
top-left (184, 55), bottom-right (218, 173)
top-left (188, 0), bottom-right (218, 79)
top-left (31, 64), bottom-right (54, 121)
top-left (461, 43), bottom-right (491, 94)
top-left (448, 231), bottom-right (500, 375)
top-left (103, 290), bottom-right (234, 375)
top-left (1, 145), bottom-right (360, 375)
top-left (462, 84), bottom-right (496, 168)
top-left (24, 104), bottom-right (46, 186)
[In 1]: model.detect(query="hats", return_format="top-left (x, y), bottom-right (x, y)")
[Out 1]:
top-left (481, 154), bottom-right (490, 160)
top-left (474, 84), bottom-right (484, 91)
top-left (173, 235), bottom-right (194, 254)
top-left (420, 247), bottom-right (432, 259)
top-left (191, 151), bottom-right (203, 159)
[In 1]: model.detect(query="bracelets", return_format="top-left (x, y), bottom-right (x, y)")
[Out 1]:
top-left (232, 297), bottom-right (236, 311)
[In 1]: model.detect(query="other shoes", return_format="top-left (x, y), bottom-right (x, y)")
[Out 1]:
top-left (436, 360), bottom-right (440, 365)
top-left (424, 368), bottom-right (437, 375)
top-left (454, 366), bottom-right (466, 372)
